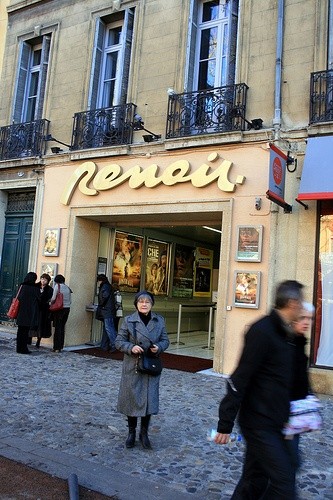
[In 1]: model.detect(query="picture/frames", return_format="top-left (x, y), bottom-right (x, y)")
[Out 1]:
top-left (41, 227), bottom-right (61, 257)
top-left (40, 262), bottom-right (57, 288)
top-left (234, 224), bottom-right (263, 263)
top-left (231, 269), bottom-right (261, 310)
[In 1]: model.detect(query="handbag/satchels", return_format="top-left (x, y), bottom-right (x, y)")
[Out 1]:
top-left (49, 293), bottom-right (63, 311)
top-left (95, 306), bottom-right (103, 322)
top-left (137, 353), bottom-right (163, 375)
top-left (7, 298), bottom-right (19, 318)
top-left (282, 395), bottom-right (323, 435)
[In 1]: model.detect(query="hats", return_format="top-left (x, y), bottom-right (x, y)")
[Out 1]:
top-left (134, 291), bottom-right (155, 308)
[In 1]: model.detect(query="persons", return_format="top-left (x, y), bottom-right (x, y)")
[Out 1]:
top-left (211, 280), bottom-right (304, 500)
top-left (149, 251), bottom-right (168, 295)
top-left (175, 244), bottom-right (195, 278)
top-left (285, 300), bottom-right (315, 466)
top-left (28, 273), bottom-right (54, 348)
top-left (16, 271), bottom-right (39, 354)
top-left (113, 232), bottom-right (142, 288)
top-left (115, 289), bottom-right (170, 450)
top-left (49, 274), bottom-right (72, 352)
top-left (95, 274), bottom-right (123, 354)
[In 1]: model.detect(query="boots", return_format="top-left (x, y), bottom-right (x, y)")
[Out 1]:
top-left (126, 416), bottom-right (137, 448)
top-left (140, 414), bottom-right (152, 449)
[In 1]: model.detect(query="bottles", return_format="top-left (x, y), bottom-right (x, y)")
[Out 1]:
top-left (205, 428), bottom-right (241, 443)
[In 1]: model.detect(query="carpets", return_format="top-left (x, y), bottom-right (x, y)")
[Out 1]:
top-left (69, 347), bottom-right (213, 373)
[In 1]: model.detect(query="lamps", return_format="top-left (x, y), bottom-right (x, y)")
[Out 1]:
top-left (132, 121), bottom-right (162, 142)
top-left (44, 134), bottom-right (72, 149)
top-left (51, 147), bottom-right (63, 154)
top-left (229, 109), bottom-right (264, 130)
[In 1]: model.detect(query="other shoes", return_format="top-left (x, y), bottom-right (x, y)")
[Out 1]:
top-left (94, 347), bottom-right (107, 352)
top-left (109, 348), bottom-right (119, 353)
top-left (50, 348), bottom-right (63, 353)
